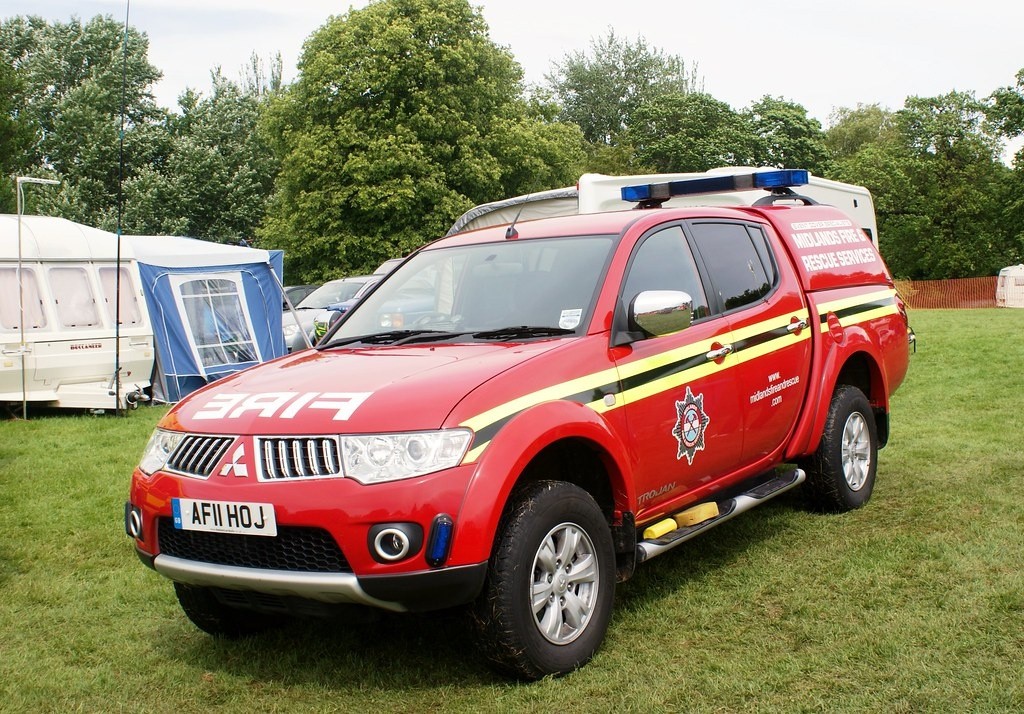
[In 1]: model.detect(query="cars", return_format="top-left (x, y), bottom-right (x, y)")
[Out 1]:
top-left (125, 169), bottom-right (909, 680)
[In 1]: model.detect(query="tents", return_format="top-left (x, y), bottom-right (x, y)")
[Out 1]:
top-left (123, 233), bottom-right (288, 405)
